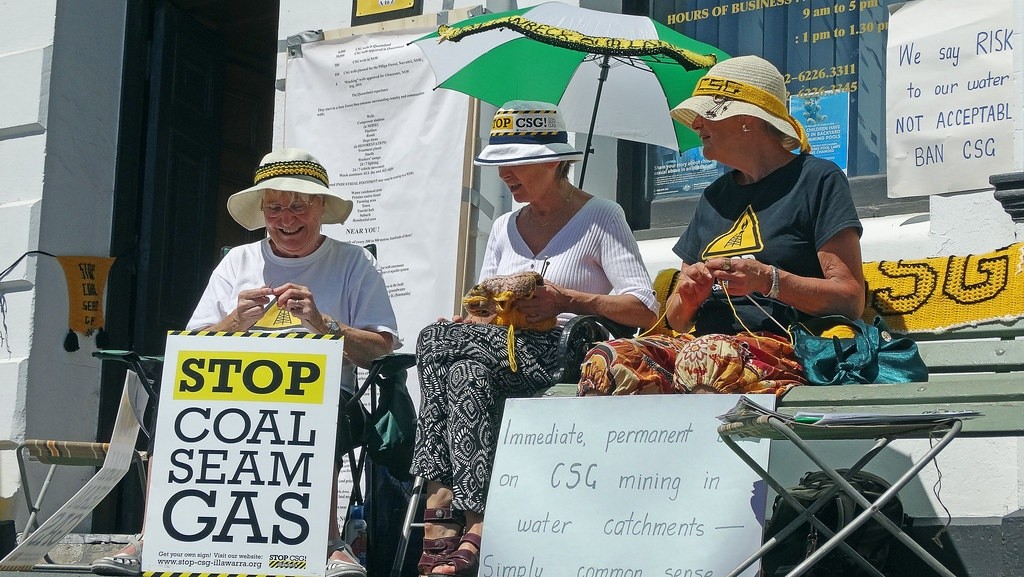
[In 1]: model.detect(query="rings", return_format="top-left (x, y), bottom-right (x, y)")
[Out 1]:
top-left (722, 280), bottom-right (729, 288)
top-left (721, 260), bottom-right (731, 270)
top-left (296, 300), bottom-right (299, 307)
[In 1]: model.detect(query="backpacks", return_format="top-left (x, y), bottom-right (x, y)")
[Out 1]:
top-left (761, 468), bottom-right (914, 577)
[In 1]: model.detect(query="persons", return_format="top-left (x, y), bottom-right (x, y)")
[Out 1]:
top-left (577, 54), bottom-right (867, 396)
top-left (409, 100), bottom-right (662, 575)
top-left (89, 149), bottom-right (405, 577)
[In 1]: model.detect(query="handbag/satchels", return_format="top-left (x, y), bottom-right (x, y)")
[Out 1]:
top-left (463, 271), bottom-right (558, 372)
top-left (790, 315), bottom-right (928, 384)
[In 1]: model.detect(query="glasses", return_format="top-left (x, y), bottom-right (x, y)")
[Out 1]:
top-left (261, 194), bottom-right (317, 217)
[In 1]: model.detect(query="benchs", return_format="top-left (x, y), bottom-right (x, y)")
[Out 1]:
top-left (533, 241), bottom-right (1024, 577)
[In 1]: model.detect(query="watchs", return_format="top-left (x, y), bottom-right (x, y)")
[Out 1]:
top-left (763, 263), bottom-right (780, 301)
top-left (327, 319), bottom-right (344, 338)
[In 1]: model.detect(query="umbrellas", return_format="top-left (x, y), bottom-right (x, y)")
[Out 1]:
top-left (405, 1), bottom-right (735, 192)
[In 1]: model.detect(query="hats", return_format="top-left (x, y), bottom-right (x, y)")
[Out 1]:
top-left (473, 100), bottom-right (584, 166)
top-left (227, 149), bottom-right (353, 231)
top-left (670, 55), bottom-right (811, 153)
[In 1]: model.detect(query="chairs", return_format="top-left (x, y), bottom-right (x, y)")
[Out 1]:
top-left (91, 243), bottom-right (416, 572)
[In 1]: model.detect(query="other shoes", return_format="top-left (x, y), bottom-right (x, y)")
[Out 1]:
top-left (325, 539), bottom-right (367, 577)
top-left (91, 534), bottom-right (144, 576)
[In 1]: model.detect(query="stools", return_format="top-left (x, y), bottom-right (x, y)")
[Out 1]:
top-left (16, 439), bottom-right (150, 570)
top-left (717, 413), bottom-right (963, 577)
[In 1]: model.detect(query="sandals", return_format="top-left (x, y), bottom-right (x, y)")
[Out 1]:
top-left (428, 532), bottom-right (481, 577)
top-left (418, 508), bottom-right (467, 577)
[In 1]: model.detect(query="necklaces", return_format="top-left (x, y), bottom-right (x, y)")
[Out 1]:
top-left (529, 186), bottom-right (577, 227)
